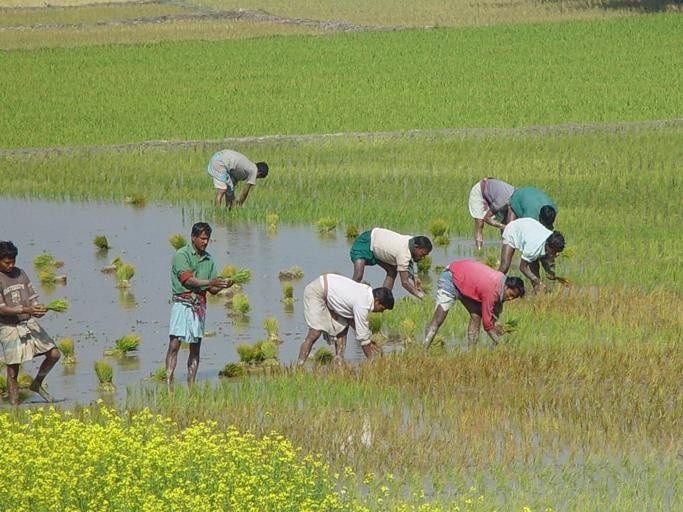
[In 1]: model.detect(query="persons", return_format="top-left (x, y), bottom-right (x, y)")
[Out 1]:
top-left (350, 226), bottom-right (432, 298)
top-left (0, 241), bottom-right (61, 410)
top-left (497, 218), bottom-right (578, 299)
top-left (421, 260), bottom-right (524, 356)
top-left (295, 273), bottom-right (395, 364)
top-left (207, 149), bottom-right (270, 210)
top-left (469, 177), bottom-right (518, 249)
top-left (507, 187), bottom-right (558, 228)
top-left (165, 222), bottom-right (233, 397)
